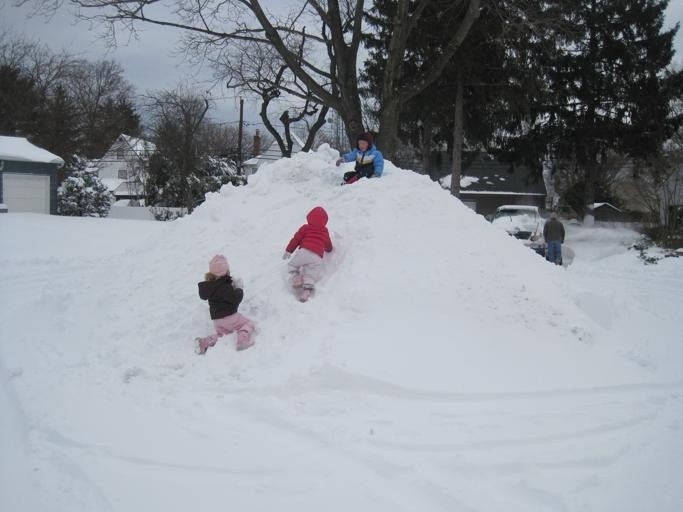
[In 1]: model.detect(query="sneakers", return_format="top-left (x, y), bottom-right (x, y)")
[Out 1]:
top-left (194, 336), bottom-right (209, 353)
top-left (237, 331), bottom-right (255, 351)
top-left (292, 276), bottom-right (310, 302)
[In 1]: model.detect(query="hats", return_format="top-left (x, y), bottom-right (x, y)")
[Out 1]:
top-left (209, 254), bottom-right (229, 277)
top-left (356, 132), bottom-right (372, 149)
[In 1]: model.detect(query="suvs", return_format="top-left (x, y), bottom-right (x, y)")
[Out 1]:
top-left (485, 203), bottom-right (546, 256)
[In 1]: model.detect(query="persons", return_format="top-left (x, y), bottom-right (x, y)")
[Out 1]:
top-left (190, 253), bottom-right (258, 354)
top-left (280, 206), bottom-right (331, 304)
top-left (544, 212), bottom-right (567, 265)
top-left (334, 136), bottom-right (387, 186)
